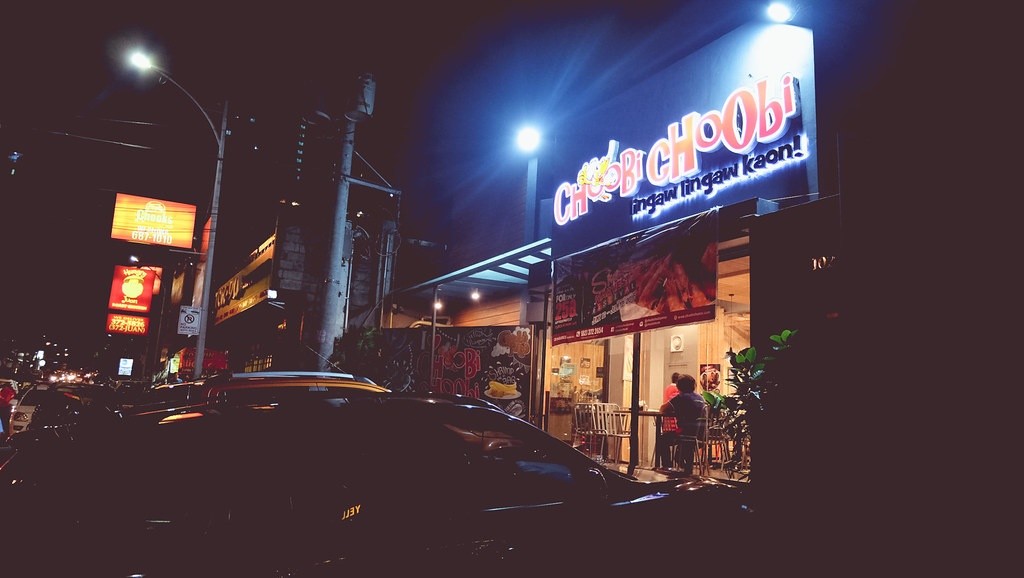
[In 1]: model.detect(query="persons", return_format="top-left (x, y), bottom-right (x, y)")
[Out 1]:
top-left (657, 374), bottom-right (707, 475)
top-left (662, 372), bottom-right (682, 433)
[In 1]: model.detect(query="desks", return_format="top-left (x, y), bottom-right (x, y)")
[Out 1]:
top-left (578, 409), bottom-right (627, 462)
top-left (613, 410), bottom-right (674, 470)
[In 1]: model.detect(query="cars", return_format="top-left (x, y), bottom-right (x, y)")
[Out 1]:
top-left (0, 371), bottom-right (746, 578)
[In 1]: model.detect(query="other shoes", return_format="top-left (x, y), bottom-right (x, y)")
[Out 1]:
top-left (660, 466), bottom-right (672, 471)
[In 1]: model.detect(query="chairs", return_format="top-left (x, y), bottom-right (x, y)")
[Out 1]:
top-left (663, 404), bottom-right (734, 479)
top-left (569, 403), bottom-right (639, 467)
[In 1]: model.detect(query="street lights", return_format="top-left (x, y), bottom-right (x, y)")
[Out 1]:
top-left (118, 40), bottom-right (231, 378)
